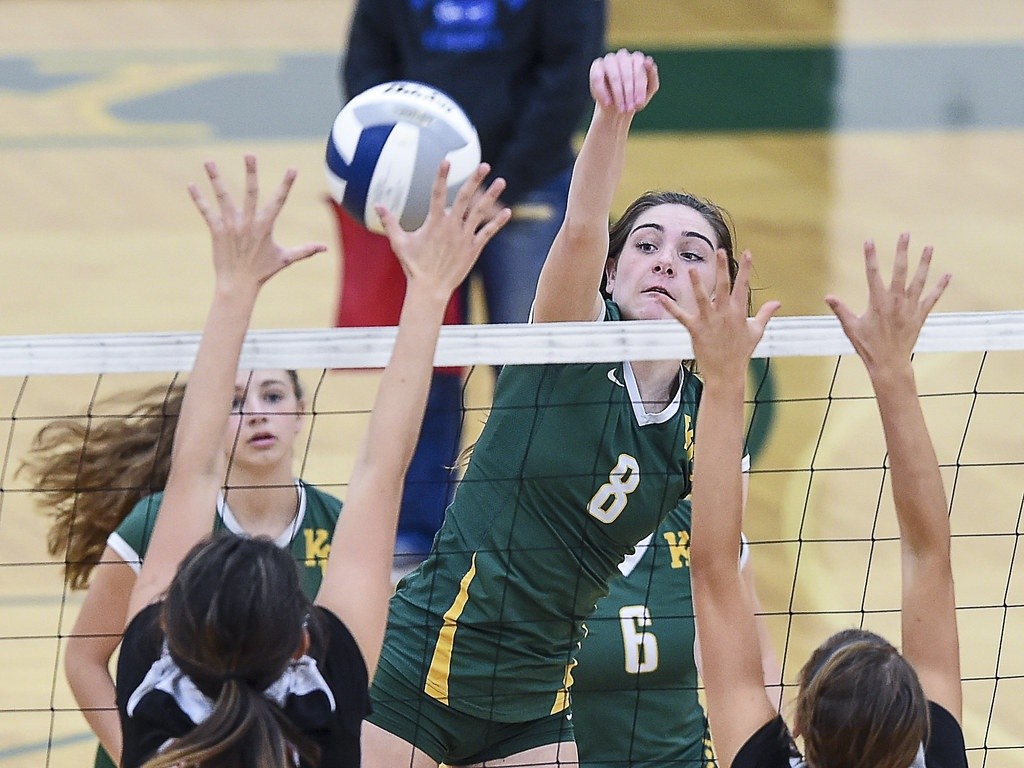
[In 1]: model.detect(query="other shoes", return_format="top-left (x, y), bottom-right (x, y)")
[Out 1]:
top-left (391, 542), bottom-right (425, 566)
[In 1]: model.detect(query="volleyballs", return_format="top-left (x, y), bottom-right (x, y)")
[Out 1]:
top-left (322, 81), bottom-right (484, 240)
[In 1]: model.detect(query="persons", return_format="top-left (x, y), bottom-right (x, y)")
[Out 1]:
top-left (340, 1), bottom-right (607, 568)
top-left (14, 363), bottom-right (342, 768)
top-left (111, 152), bottom-right (513, 768)
top-left (361, 46), bottom-right (968, 768)
